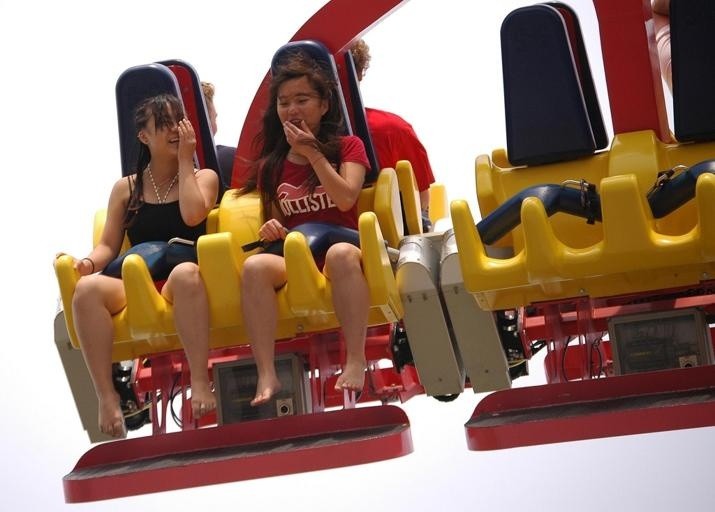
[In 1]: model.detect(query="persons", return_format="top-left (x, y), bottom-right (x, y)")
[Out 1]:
top-left (337, 37), bottom-right (438, 241)
top-left (56, 92), bottom-right (225, 440)
top-left (239, 55), bottom-right (372, 404)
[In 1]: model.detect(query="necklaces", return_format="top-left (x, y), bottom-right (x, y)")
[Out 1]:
top-left (146, 162), bottom-right (181, 205)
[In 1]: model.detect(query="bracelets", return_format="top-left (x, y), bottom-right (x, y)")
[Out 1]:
top-left (80, 258), bottom-right (94, 275)
